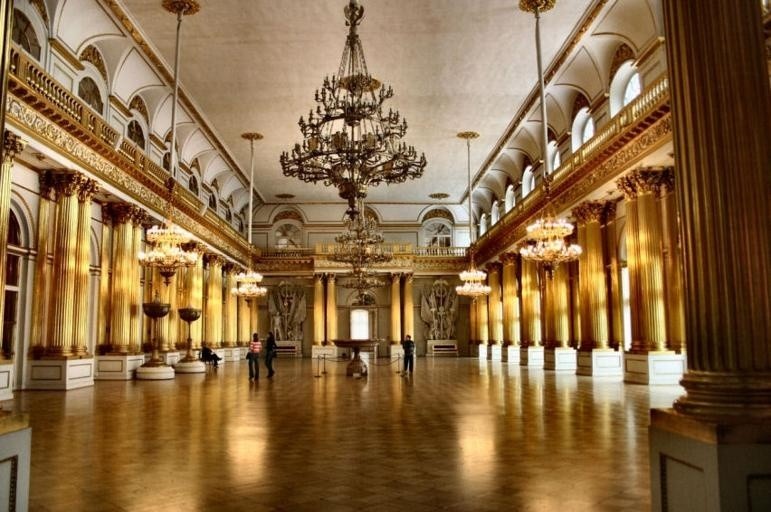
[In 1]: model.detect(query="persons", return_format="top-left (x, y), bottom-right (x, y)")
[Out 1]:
top-left (265, 332), bottom-right (275, 378)
top-left (249, 377), bottom-right (274, 392)
top-left (203, 367), bottom-right (218, 386)
top-left (424, 322), bottom-right (436, 340)
top-left (202, 340), bottom-right (222, 368)
top-left (246, 333), bottom-right (263, 381)
top-left (403, 335), bottom-right (414, 373)
top-left (401, 373), bottom-right (414, 401)
top-left (270, 312), bottom-right (283, 341)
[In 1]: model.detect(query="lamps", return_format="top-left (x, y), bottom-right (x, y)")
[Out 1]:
top-left (138, 0), bottom-right (202, 288)
top-left (518, 0), bottom-right (586, 280)
top-left (325, 197), bottom-right (394, 304)
top-left (279, 1), bottom-right (430, 221)
top-left (231, 131), bottom-right (268, 310)
top-left (456, 129), bottom-right (495, 306)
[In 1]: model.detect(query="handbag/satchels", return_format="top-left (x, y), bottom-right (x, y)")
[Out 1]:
top-left (246, 352), bottom-right (252, 360)
top-left (273, 351), bottom-right (278, 359)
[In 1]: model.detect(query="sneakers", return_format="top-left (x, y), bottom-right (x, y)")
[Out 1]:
top-left (249, 376), bottom-right (259, 382)
top-left (267, 370), bottom-right (275, 378)
top-left (213, 365), bottom-right (219, 368)
top-left (217, 357), bottom-right (222, 361)
